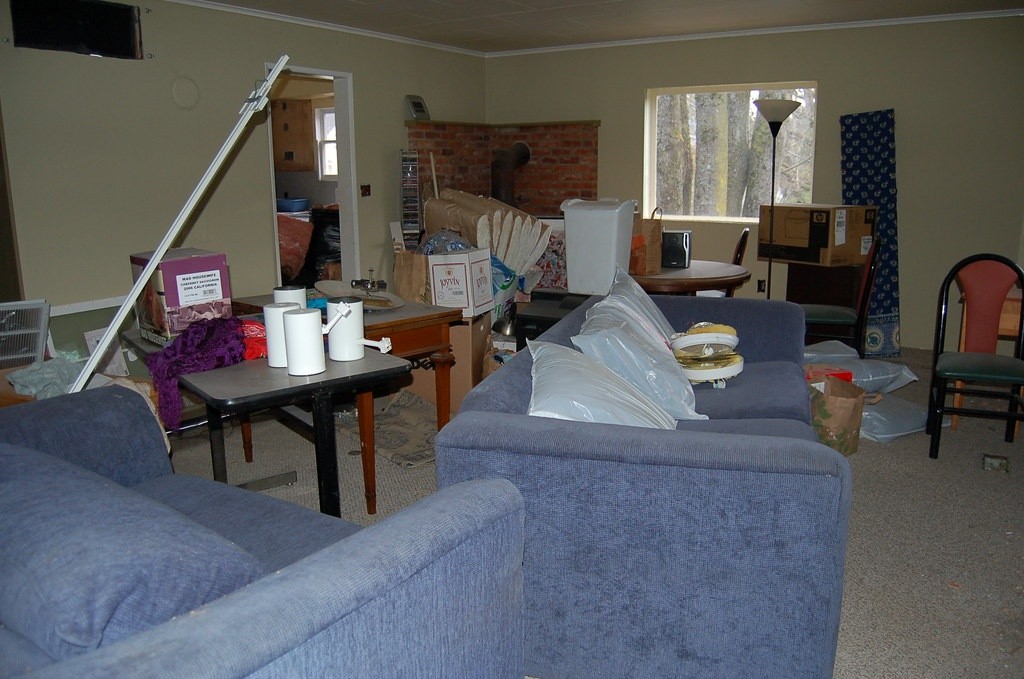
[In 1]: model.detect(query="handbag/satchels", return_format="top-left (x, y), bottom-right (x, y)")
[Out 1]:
top-left (805, 367), bottom-right (882, 456)
top-left (633, 207), bottom-right (662, 274)
top-left (393, 241), bottom-right (432, 306)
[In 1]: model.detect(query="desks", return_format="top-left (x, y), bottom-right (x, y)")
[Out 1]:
top-left (230, 291), bottom-right (463, 516)
top-left (122, 312), bottom-right (414, 519)
top-left (629, 259), bottom-right (752, 297)
top-left (950, 289), bottom-right (1024, 438)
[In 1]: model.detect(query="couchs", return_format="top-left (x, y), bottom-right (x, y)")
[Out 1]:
top-left (0, 384), bottom-right (527, 679)
top-left (432, 264), bottom-right (852, 679)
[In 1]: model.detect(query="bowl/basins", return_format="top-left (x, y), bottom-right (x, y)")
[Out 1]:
top-left (277, 198), bottom-right (310, 211)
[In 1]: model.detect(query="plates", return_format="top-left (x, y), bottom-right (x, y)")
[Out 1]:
top-left (314, 280), bottom-right (406, 311)
top-left (670, 333), bottom-right (740, 355)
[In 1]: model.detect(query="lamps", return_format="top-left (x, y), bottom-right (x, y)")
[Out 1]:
top-left (753, 100), bottom-right (800, 300)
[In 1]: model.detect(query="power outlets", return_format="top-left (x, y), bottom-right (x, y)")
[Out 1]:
top-left (757, 280), bottom-right (766, 293)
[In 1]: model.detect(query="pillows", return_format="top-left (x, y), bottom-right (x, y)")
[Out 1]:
top-left (859, 394), bottom-right (952, 443)
top-left (525, 337), bottom-right (679, 430)
top-left (0, 442), bottom-right (270, 663)
top-left (829, 359), bottom-right (919, 394)
top-left (570, 265), bottom-right (710, 421)
top-left (804, 340), bottom-right (860, 358)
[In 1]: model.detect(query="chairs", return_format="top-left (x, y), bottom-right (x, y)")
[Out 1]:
top-left (926, 252), bottom-right (1024, 459)
top-left (696, 227), bottom-right (749, 297)
top-left (800, 233), bottom-right (882, 359)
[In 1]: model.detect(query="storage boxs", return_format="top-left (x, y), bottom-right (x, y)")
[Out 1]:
top-left (130, 247), bottom-right (233, 348)
top-left (428, 247), bottom-right (496, 318)
top-left (449, 312), bottom-right (491, 412)
top-left (757, 203), bottom-right (878, 267)
